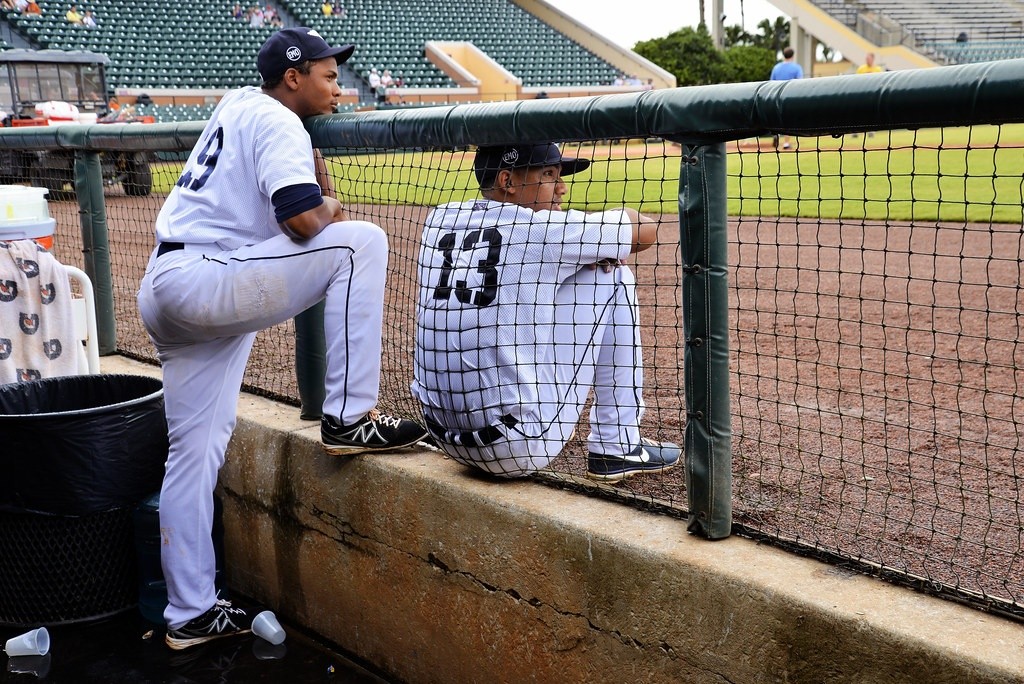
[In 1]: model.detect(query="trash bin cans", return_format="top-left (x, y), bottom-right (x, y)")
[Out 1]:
top-left (0, 372), bottom-right (171, 634)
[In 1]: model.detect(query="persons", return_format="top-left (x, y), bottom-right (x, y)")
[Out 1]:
top-left (368, 67), bottom-right (383, 98)
top-left (768, 46), bottom-right (805, 148)
top-left (0, 0), bottom-right (42, 14)
top-left (231, 3), bottom-right (284, 30)
top-left (381, 69), bottom-right (397, 89)
top-left (614, 72), bottom-right (654, 91)
top-left (412, 142), bottom-right (683, 484)
top-left (853, 52), bottom-right (885, 137)
top-left (3, 101), bottom-right (36, 124)
top-left (133, 26), bottom-right (433, 650)
top-left (322, 0), bottom-right (343, 17)
top-left (66, 3), bottom-right (97, 27)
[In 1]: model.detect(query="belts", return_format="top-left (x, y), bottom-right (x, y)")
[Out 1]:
top-left (158, 242), bottom-right (185, 255)
top-left (424, 415), bottom-right (518, 447)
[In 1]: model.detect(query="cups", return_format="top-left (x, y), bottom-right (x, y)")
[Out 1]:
top-left (5, 627), bottom-right (50, 656)
top-left (251, 610), bottom-right (286, 645)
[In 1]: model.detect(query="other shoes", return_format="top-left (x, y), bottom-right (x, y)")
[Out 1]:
top-left (772, 136), bottom-right (779, 147)
top-left (783, 144), bottom-right (792, 149)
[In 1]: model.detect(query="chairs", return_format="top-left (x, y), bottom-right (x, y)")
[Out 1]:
top-left (810, 0), bottom-right (1024, 66)
top-left (1, 0), bottom-right (651, 161)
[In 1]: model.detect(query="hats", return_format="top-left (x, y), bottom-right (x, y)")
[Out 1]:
top-left (475, 143), bottom-right (590, 185)
top-left (257, 27), bottom-right (355, 81)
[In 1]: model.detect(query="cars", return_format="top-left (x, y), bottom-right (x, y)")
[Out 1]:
top-left (0, 48), bottom-right (153, 200)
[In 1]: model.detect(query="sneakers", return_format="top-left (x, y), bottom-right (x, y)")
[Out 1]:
top-left (165, 591), bottom-right (259, 650)
top-left (321, 409), bottom-right (429, 455)
top-left (589, 439), bottom-right (681, 480)
